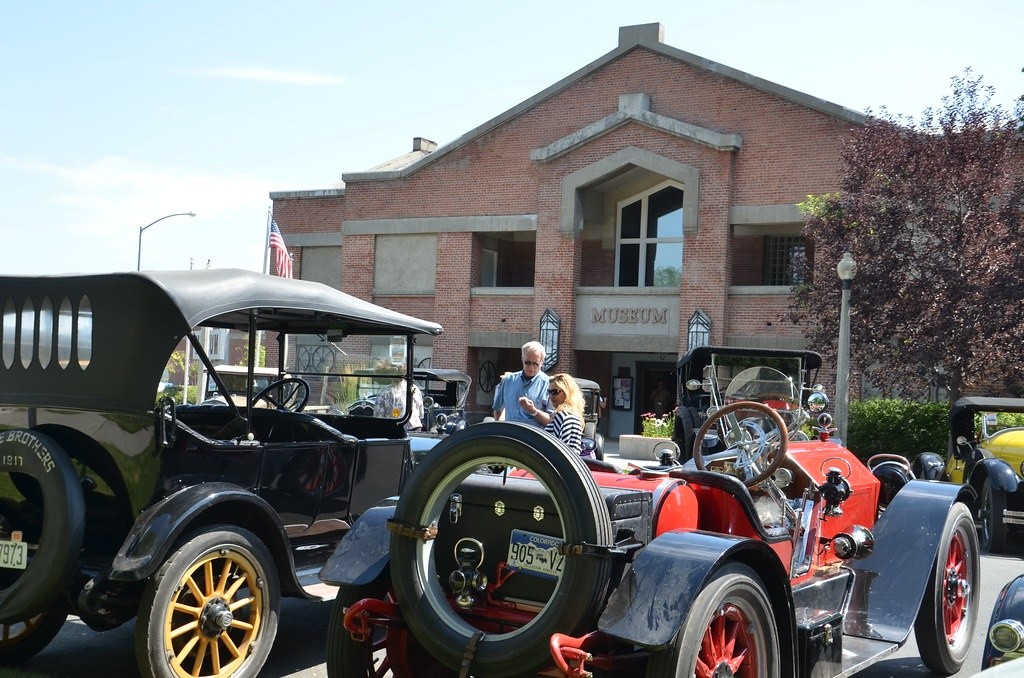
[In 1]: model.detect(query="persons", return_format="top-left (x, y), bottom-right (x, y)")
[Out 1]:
top-left (543, 373), bottom-right (586, 456)
top-left (492, 341), bottom-right (556, 430)
top-left (650, 380), bottom-right (675, 417)
top-left (372, 356), bottom-right (425, 433)
top-left (596, 395), bottom-right (607, 430)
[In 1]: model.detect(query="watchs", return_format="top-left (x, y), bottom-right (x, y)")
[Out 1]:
top-left (530, 409), bottom-right (539, 417)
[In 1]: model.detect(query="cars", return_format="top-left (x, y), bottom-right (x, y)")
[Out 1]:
top-left (317, 402), bottom-right (980, 678)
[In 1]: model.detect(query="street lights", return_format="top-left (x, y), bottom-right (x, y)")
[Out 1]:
top-left (136, 212), bottom-right (197, 268)
top-left (831, 251), bottom-right (860, 448)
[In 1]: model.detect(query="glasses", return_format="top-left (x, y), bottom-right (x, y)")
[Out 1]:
top-left (547, 389), bottom-right (563, 395)
top-left (523, 361), bottom-right (541, 366)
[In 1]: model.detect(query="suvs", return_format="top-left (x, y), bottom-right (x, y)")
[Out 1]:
top-left (567, 377), bottom-right (608, 463)
top-left (201, 365), bottom-right (292, 408)
top-left (668, 347), bottom-right (826, 466)
top-left (346, 366), bottom-right (472, 436)
top-left (1, 267), bottom-right (464, 678)
top-left (904, 396), bottom-right (1024, 553)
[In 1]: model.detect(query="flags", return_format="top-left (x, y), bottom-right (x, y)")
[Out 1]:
top-left (269, 213), bottom-right (293, 279)
